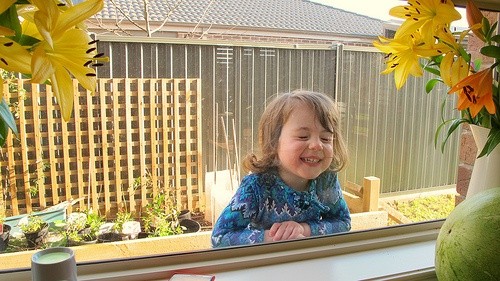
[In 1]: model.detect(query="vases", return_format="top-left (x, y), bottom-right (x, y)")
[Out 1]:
top-left (467, 123), bottom-right (500, 200)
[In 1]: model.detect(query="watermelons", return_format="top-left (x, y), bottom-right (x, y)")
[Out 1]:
top-left (434, 186), bottom-right (500, 281)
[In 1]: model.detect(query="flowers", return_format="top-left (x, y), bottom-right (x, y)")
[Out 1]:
top-left (0, 0), bottom-right (110, 147)
top-left (372, 0), bottom-right (500, 159)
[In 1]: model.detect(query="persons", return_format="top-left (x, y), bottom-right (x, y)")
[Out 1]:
top-left (211, 90), bottom-right (352, 248)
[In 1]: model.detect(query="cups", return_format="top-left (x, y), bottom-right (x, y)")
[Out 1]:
top-left (30, 246), bottom-right (80, 281)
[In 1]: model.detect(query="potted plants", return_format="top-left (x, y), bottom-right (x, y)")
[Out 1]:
top-left (0, 168), bottom-right (202, 249)
